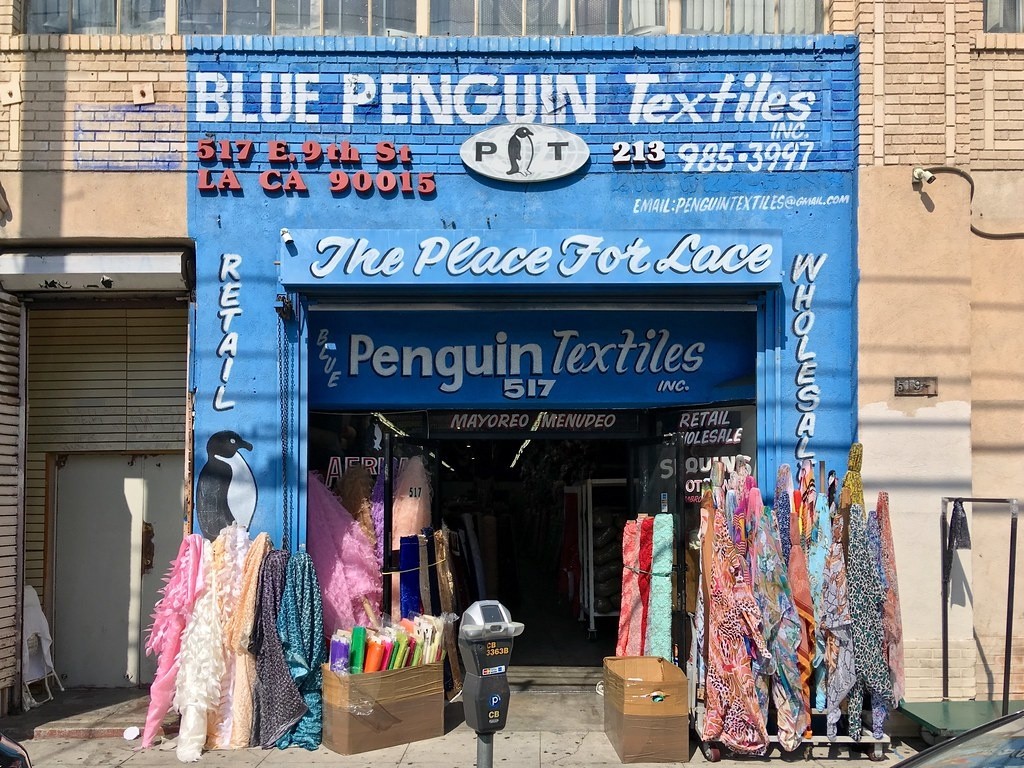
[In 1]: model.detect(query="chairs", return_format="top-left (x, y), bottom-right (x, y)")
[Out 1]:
top-left (21, 585), bottom-right (64, 712)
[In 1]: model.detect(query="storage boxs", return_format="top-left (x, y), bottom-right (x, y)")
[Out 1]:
top-left (320, 662), bottom-right (443, 756)
top-left (602, 655), bottom-right (689, 764)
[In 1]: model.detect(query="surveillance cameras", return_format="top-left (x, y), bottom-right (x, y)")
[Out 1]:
top-left (916, 170), bottom-right (936, 184)
top-left (281, 230), bottom-right (293, 244)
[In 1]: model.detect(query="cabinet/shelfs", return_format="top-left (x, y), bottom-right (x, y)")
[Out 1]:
top-left (563, 476), bottom-right (640, 645)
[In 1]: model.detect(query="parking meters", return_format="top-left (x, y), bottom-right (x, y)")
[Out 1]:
top-left (458, 599), bottom-right (524, 768)
